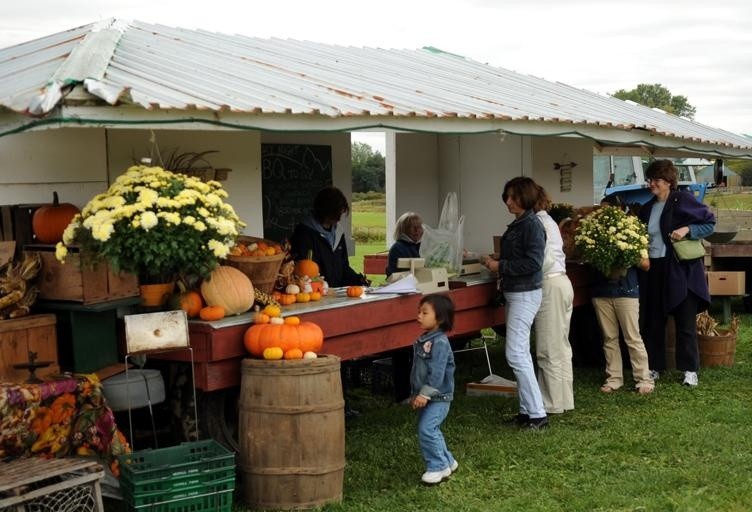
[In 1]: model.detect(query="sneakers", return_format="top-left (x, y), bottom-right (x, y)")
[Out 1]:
top-left (600, 370), bottom-right (661, 394)
top-left (681, 370), bottom-right (699, 386)
top-left (421, 461), bottom-right (459, 485)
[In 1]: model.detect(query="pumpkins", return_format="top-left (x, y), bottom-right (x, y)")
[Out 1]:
top-left (347, 285), bottom-right (363, 297)
top-left (172, 236), bottom-right (328, 360)
top-left (33, 188), bottom-right (80, 244)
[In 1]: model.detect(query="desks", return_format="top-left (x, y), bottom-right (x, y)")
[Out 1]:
top-left (1, 455), bottom-right (106, 512)
top-left (153, 255), bottom-right (506, 393)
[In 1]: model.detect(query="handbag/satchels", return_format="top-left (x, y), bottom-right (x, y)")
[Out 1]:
top-left (667, 232), bottom-right (707, 261)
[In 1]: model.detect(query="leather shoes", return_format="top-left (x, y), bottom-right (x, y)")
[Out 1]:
top-left (502, 412), bottom-right (548, 431)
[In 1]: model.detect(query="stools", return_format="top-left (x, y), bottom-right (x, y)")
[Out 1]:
top-left (100, 368), bottom-right (166, 447)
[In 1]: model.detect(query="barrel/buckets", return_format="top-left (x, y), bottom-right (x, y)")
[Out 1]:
top-left (236, 353), bottom-right (346, 511)
top-left (218, 234), bottom-right (290, 296)
top-left (101, 368), bottom-right (173, 452)
top-left (697, 329), bottom-right (737, 368)
top-left (663, 332), bottom-right (678, 369)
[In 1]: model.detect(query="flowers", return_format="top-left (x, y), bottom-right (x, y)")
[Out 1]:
top-left (56, 165), bottom-right (246, 277)
top-left (573, 206), bottom-right (649, 267)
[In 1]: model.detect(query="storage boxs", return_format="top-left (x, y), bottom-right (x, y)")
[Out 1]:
top-left (0, 204), bottom-right (42, 266)
top-left (707, 270), bottom-right (745, 295)
top-left (0, 312), bottom-right (59, 382)
top-left (22, 238), bottom-right (139, 304)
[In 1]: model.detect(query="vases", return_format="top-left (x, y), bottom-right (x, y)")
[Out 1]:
top-left (140, 276), bottom-right (173, 307)
top-left (599, 261), bottom-right (624, 282)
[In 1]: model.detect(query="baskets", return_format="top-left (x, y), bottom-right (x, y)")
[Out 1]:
top-left (118, 438), bottom-right (237, 512)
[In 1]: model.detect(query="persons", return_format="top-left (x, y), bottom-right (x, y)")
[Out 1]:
top-left (480, 176), bottom-right (547, 429)
top-left (637, 160), bottom-right (716, 386)
top-left (384, 211), bottom-right (425, 282)
top-left (408, 291), bottom-right (459, 485)
top-left (590, 195), bottom-right (656, 393)
top-left (289, 186), bottom-right (372, 288)
top-left (491, 184), bottom-right (574, 416)
